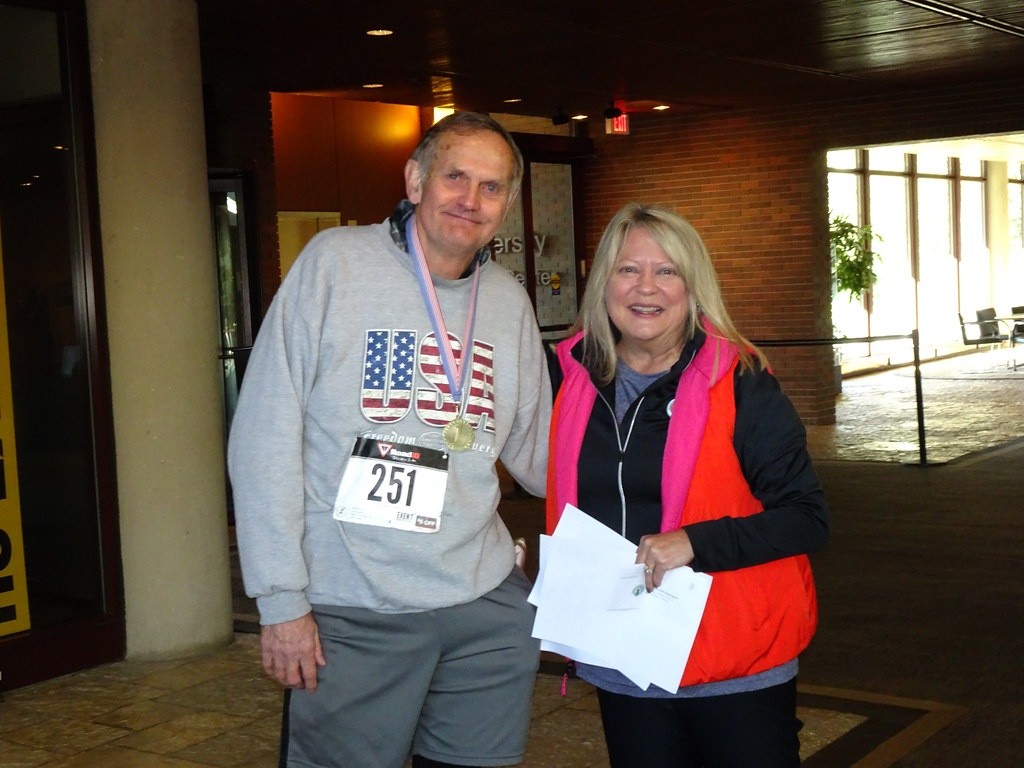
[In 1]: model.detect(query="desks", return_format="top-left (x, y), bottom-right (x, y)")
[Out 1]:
top-left (995, 313), bottom-right (1024, 371)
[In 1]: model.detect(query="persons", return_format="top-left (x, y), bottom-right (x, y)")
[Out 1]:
top-left (510, 203), bottom-right (828, 768)
top-left (227, 111), bottom-right (563, 768)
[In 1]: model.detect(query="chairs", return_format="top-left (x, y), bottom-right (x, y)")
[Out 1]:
top-left (956, 313), bottom-right (1008, 373)
top-left (1012, 306), bottom-right (1024, 372)
top-left (976, 308), bottom-right (1011, 370)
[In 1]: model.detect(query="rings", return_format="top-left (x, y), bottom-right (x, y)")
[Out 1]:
top-left (644, 565), bottom-right (654, 573)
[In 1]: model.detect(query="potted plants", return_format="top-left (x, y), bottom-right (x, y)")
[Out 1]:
top-left (828, 211), bottom-right (886, 396)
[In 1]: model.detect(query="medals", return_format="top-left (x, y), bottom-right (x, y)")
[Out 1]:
top-left (443, 419), bottom-right (476, 452)
